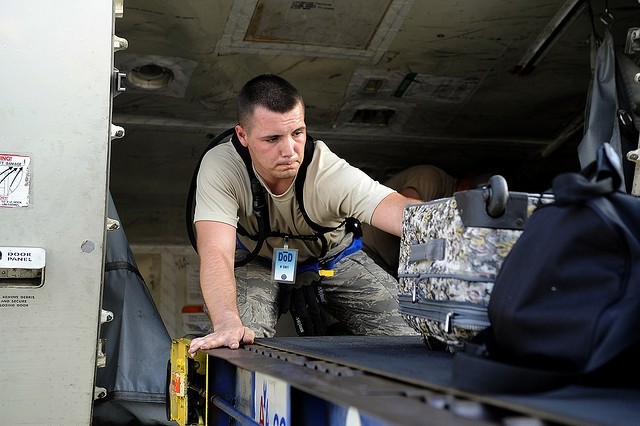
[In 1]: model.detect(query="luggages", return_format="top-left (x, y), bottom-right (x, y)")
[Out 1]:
top-left (397, 176), bottom-right (555, 354)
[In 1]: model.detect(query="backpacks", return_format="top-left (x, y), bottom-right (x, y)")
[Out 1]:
top-left (453, 142), bottom-right (640, 393)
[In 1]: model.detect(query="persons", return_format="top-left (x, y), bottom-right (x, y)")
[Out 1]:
top-left (187, 72), bottom-right (426, 359)
top-left (289, 164), bottom-right (477, 338)
